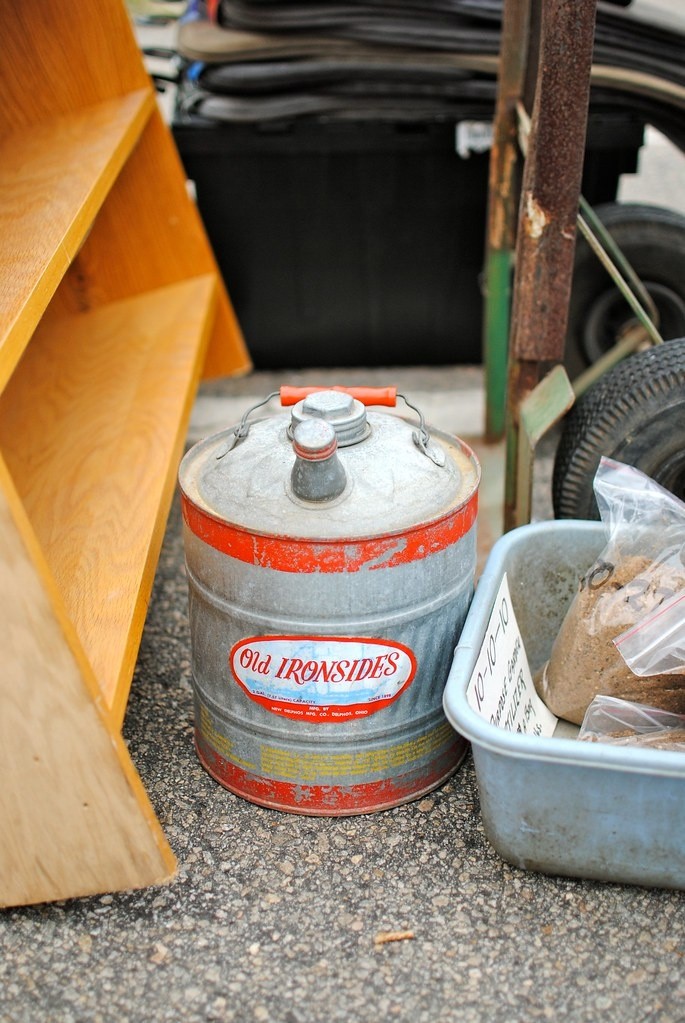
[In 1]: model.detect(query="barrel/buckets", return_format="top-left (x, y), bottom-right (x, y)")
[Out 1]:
top-left (177, 383), bottom-right (482, 816)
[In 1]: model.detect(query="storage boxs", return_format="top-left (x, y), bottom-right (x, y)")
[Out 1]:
top-left (441, 520), bottom-right (685, 895)
